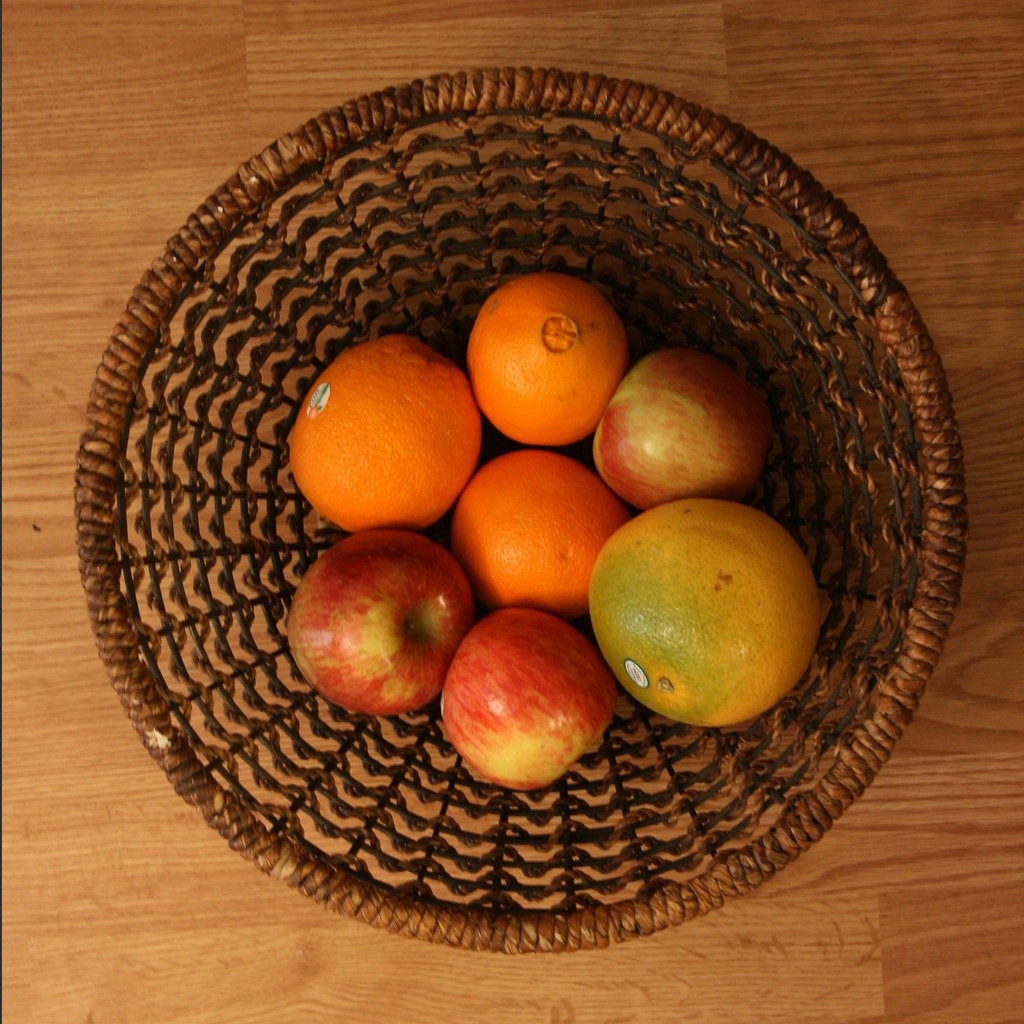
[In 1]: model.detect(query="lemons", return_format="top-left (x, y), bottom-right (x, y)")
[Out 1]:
top-left (589, 498), bottom-right (822, 728)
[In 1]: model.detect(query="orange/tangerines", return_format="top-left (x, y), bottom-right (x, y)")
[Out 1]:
top-left (288, 271), bottom-right (639, 620)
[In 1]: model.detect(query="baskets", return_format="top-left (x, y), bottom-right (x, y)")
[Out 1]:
top-left (72, 67), bottom-right (968, 954)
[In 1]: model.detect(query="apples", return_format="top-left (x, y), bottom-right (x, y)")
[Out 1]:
top-left (284, 347), bottom-right (769, 794)
